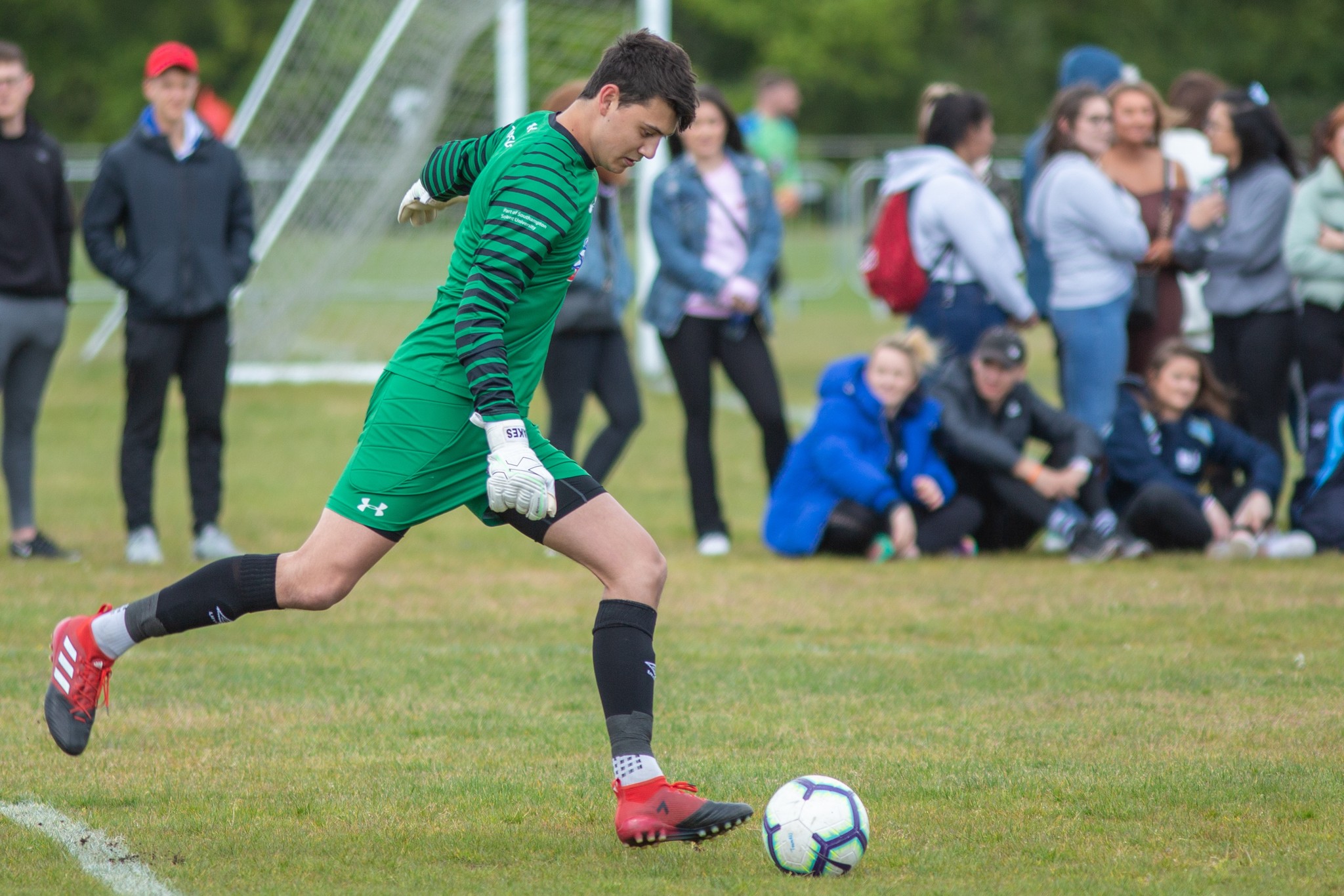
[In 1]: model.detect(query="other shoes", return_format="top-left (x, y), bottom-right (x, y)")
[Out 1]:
top-left (1067, 523), bottom-right (1153, 560)
top-left (699, 532), bottom-right (731, 558)
top-left (10, 530), bottom-right (77, 559)
top-left (1204, 528), bottom-right (1316, 560)
top-left (123, 524), bottom-right (162, 565)
top-left (961, 536), bottom-right (981, 555)
top-left (871, 534), bottom-right (921, 560)
top-left (192, 522), bottom-right (240, 561)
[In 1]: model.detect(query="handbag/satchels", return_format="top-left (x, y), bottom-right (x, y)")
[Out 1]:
top-left (1132, 270), bottom-right (1161, 319)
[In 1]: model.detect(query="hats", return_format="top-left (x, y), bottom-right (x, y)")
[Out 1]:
top-left (977, 330), bottom-right (1025, 367)
top-left (145, 40), bottom-right (200, 78)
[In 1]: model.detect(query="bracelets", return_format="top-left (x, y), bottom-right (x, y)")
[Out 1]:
top-left (1024, 462), bottom-right (1046, 488)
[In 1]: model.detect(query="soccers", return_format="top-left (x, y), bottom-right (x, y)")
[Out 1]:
top-left (762, 774), bottom-right (869, 877)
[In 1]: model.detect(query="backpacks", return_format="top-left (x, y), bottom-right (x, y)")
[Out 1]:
top-left (1286, 384), bottom-right (1344, 547)
top-left (862, 181), bottom-right (953, 317)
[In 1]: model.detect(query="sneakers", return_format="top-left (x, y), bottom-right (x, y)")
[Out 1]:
top-left (609, 776), bottom-right (756, 849)
top-left (44, 603), bottom-right (115, 756)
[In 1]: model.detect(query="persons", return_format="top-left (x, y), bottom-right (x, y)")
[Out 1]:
top-left (739, 83), bottom-right (825, 223)
top-left (536, 80), bottom-right (641, 487)
top-left (0, 42), bottom-right (71, 565)
top-left (44, 27), bottom-right (754, 852)
top-left (761, 44), bottom-right (1344, 560)
top-left (646, 88), bottom-right (792, 560)
top-left (82, 41), bottom-right (245, 568)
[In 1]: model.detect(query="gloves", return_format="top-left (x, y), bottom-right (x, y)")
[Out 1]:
top-left (468, 413), bottom-right (557, 522)
top-left (397, 178), bottom-right (468, 227)
